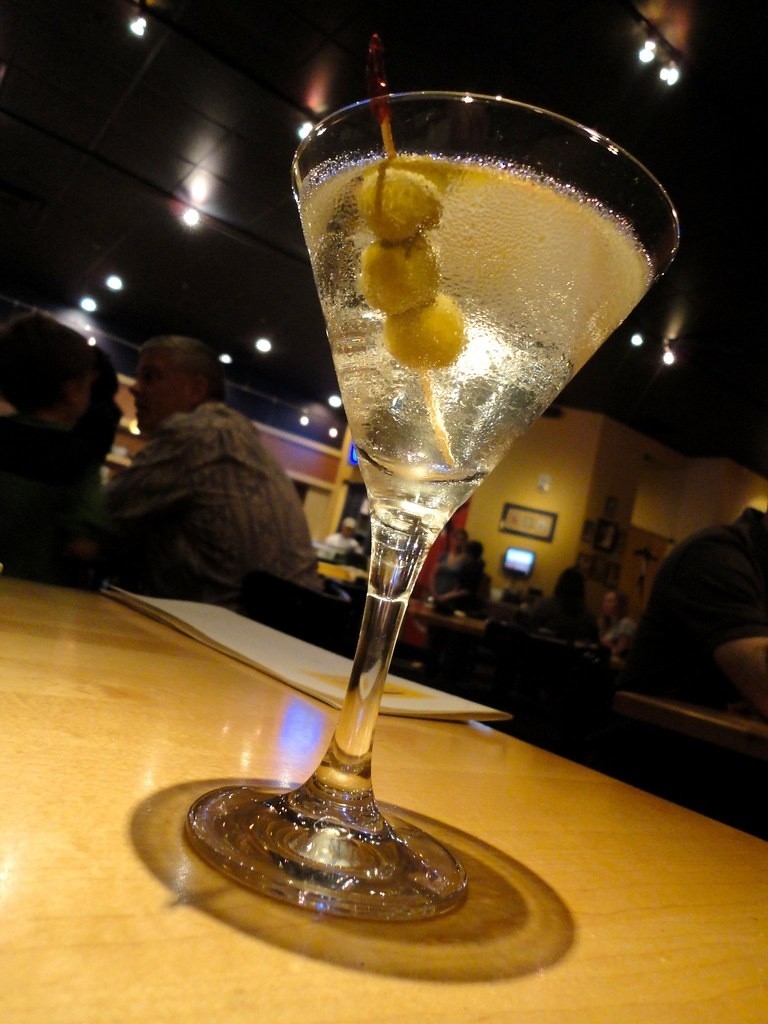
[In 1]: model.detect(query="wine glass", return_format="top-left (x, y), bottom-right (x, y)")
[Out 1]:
top-left (188, 91), bottom-right (680, 923)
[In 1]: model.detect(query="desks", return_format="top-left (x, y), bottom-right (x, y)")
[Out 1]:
top-left (408, 598), bottom-right (504, 639)
top-left (613, 690), bottom-right (768, 761)
top-left (1, 576), bottom-right (768, 1024)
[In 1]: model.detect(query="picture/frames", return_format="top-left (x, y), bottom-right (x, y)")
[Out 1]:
top-left (498, 501), bottom-right (558, 543)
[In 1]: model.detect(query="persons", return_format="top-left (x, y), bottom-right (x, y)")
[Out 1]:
top-left (1, 307), bottom-right (365, 611)
top-left (426, 507), bottom-right (768, 844)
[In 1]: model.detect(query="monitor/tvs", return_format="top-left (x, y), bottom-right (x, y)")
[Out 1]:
top-left (503, 547), bottom-right (535, 575)
